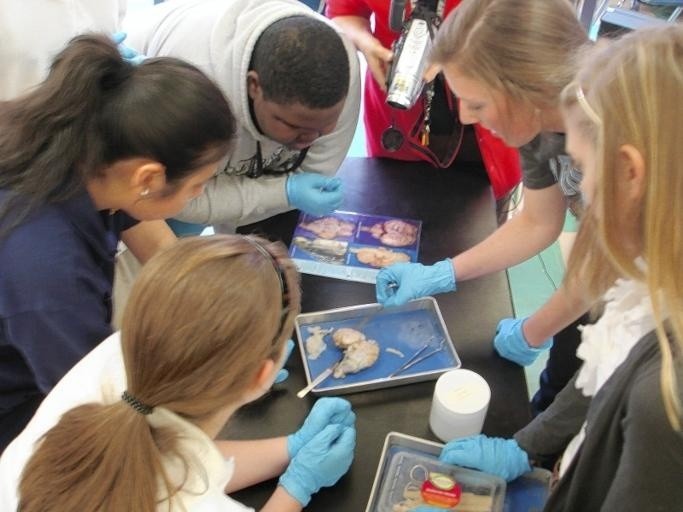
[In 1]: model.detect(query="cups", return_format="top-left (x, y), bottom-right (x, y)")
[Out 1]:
top-left (428, 368), bottom-right (491, 443)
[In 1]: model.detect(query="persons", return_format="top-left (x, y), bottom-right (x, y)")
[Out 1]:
top-left (324, 0), bottom-right (522, 229)
top-left (119, 0), bottom-right (361, 247)
top-left (0, 234), bottom-right (356, 512)
top-left (436, 24), bottom-right (683, 512)
top-left (376, 0), bottom-right (642, 482)
top-left (0, 35), bottom-right (238, 459)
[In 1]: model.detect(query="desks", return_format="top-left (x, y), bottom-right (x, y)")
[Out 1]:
top-left (215, 154), bottom-right (537, 512)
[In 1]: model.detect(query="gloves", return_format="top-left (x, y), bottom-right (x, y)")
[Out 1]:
top-left (374, 256), bottom-right (458, 310)
top-left (285, 396), bottom-right (357, 469)
top-left (437, 433), bottom-right (534, 486)
top-left (492, 316), bottom-right (556, 368)
top-left (285, 170), bottom-right (345, 218)
top-left (276, 423), bottom-right (357, 508)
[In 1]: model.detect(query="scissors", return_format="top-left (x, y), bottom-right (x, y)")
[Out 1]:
top-left (404, 465), bottom-right (428, 497)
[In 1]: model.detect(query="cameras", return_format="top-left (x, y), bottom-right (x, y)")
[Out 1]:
top-left (385, 18), bottom-right (436, 110)
